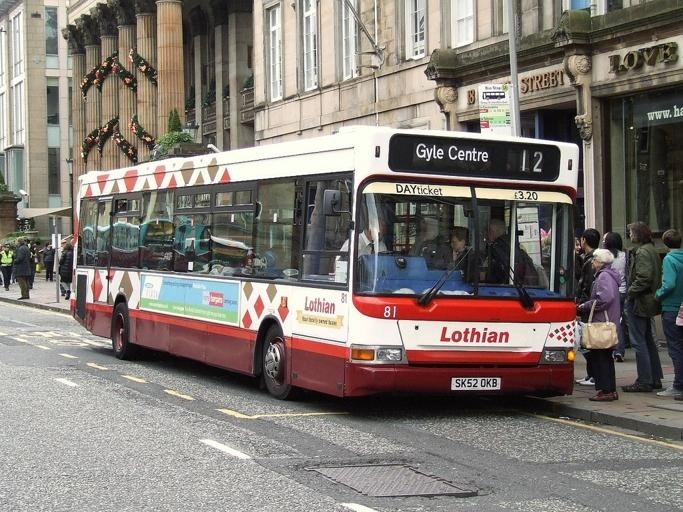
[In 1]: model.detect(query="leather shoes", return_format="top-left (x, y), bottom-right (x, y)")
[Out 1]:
top-left (589, 391), bottom-right (614, 401)
top-left (612, 391), bottom-right (618, 400)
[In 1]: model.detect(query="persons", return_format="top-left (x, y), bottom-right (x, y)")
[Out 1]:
top-left (448, 227), bottom-right (483, 281)
top-left (485, 215), bottom-right (524, 285)
top-left (334, 217), bottom-right (389, 286)
top-left (575, 222), bottom-right (683, 401)
top-left (0, 237), bottom-right (72, 303)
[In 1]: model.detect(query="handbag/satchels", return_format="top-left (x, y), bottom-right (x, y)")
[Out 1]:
top-left (578, 300), bottom-right (620, 350)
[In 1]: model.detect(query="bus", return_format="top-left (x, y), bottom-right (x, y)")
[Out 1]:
top-left (68, 121), bottom-right (590, 401)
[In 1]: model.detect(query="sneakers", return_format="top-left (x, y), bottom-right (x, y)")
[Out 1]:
top-left (576, 376), bottom-right (591, 384)
top-left (580, 377), bottom-right (595, 386)
top-left (657, 385), bottom-right (683, 397)
top-left (615, 351), bottom-right (624, 362)
top-left (650, 379), bottom-right (662, 389)
top-left (64, 290), bottom-right (71, 300)
top-left (620, 381), bottom-right (653, 392)
